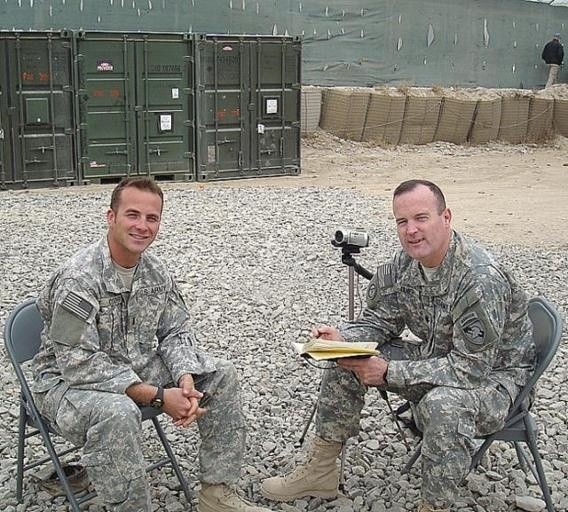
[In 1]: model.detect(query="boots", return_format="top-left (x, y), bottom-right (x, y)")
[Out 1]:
top-left (256, 434), bottom-right (344, 501)
top-left (196, 482), bottom-right (274, 512)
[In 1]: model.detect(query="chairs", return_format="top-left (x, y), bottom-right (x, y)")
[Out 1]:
top-left (402, 297), bottom-right (562, 511)
top-left (3, 299), bottom-right (193, 512)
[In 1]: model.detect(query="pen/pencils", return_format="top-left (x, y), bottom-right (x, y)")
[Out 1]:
top-left (316, 334), bottom-right (322, 339)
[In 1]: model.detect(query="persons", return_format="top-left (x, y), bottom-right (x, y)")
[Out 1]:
top-left (34, 175), bottom-right (268, 512)
top-left (541, 32), bottom-right (564, 90)
top-left (261, 179), bottom-right (537, 512)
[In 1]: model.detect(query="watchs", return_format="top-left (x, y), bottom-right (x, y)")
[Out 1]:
top-left (151, 385), bottom-right (164, 410)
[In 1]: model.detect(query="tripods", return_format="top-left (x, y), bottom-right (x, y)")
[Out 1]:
top-left (299, 247), bottom-right (412, 452)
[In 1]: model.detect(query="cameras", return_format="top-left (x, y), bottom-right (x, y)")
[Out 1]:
top-left (330, 227), bottom-right (370, 247)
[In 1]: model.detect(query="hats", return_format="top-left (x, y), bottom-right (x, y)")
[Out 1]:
top-left (29, 459), bottom-right (92, 496)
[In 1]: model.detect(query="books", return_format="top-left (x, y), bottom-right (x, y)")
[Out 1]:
top-left (290, 337), bottom-right (380, 363)
